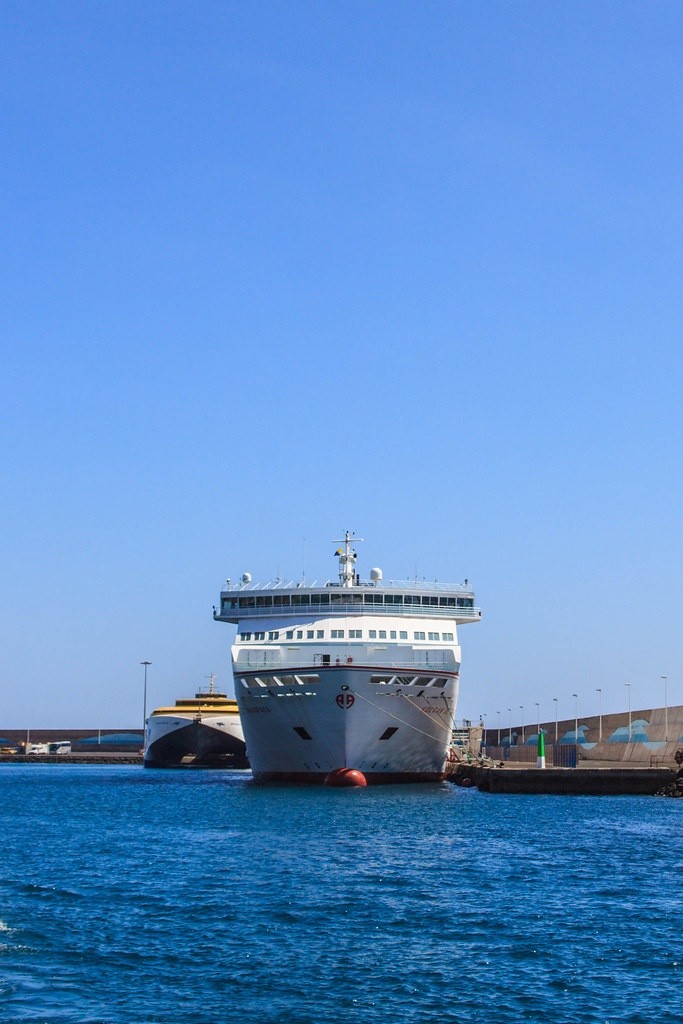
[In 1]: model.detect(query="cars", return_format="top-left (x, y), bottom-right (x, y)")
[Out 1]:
top-left (28, 743), bottom-right (48, 755)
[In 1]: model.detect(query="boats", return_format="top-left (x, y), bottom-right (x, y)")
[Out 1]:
top-left (144, 674), bottom-right (250, 769)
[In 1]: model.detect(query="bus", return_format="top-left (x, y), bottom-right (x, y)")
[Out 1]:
top-left (48, 740), bottom-right (72, 755)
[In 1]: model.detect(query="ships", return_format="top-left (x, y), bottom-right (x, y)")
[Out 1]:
top-left (212, 529), bottom-right (482, 786)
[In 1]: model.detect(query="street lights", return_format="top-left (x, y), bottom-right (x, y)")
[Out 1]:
top-left (508, 708), bottom-right (511, 745)
top-left (535, 703), bottom-right (540, 735)
top-left (484, 713), bottom-right (486, 747)
top-left (596, 688), bottom-right (602, 743)
top-left (140, 661), bottom-right (152, 755)
top-left (624, 683), bottom-right (632, 743)
top-left (520, 705), bottom-right (524, 744)
top-left (572, 693), bottom-right (578, 744)
top-left (497, 711), bottom-right (500, 746)
top-left (660, 675), bottom-right (668, 743)
top-left (553, 698), bottom-right (558, 745)
